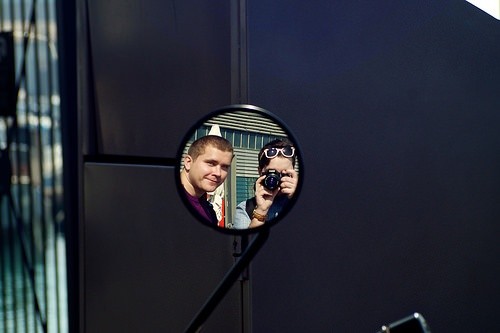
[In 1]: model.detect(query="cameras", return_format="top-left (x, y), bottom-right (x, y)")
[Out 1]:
top-left (262, 169), bottom-right (291, 189)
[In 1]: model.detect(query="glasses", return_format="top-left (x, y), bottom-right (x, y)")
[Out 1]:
top-left (260, 147), bottom-right (296, 159)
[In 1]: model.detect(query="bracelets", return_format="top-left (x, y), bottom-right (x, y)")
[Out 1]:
top-left (253, 205), bottom-right (268, 223)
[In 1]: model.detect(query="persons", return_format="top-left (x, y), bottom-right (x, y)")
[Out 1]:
top-left (179, 134), bottom-right (235, 227)
top-left (233, 138), bottom-right (299, 230)
